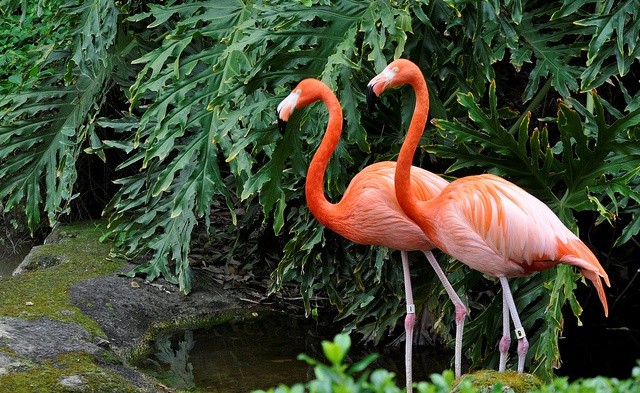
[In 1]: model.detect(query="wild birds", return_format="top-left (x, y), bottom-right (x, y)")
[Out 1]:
top-left (276, 78), bottom-right (468, 393)
top-left (367, 59), bottom-right (612, 374)
